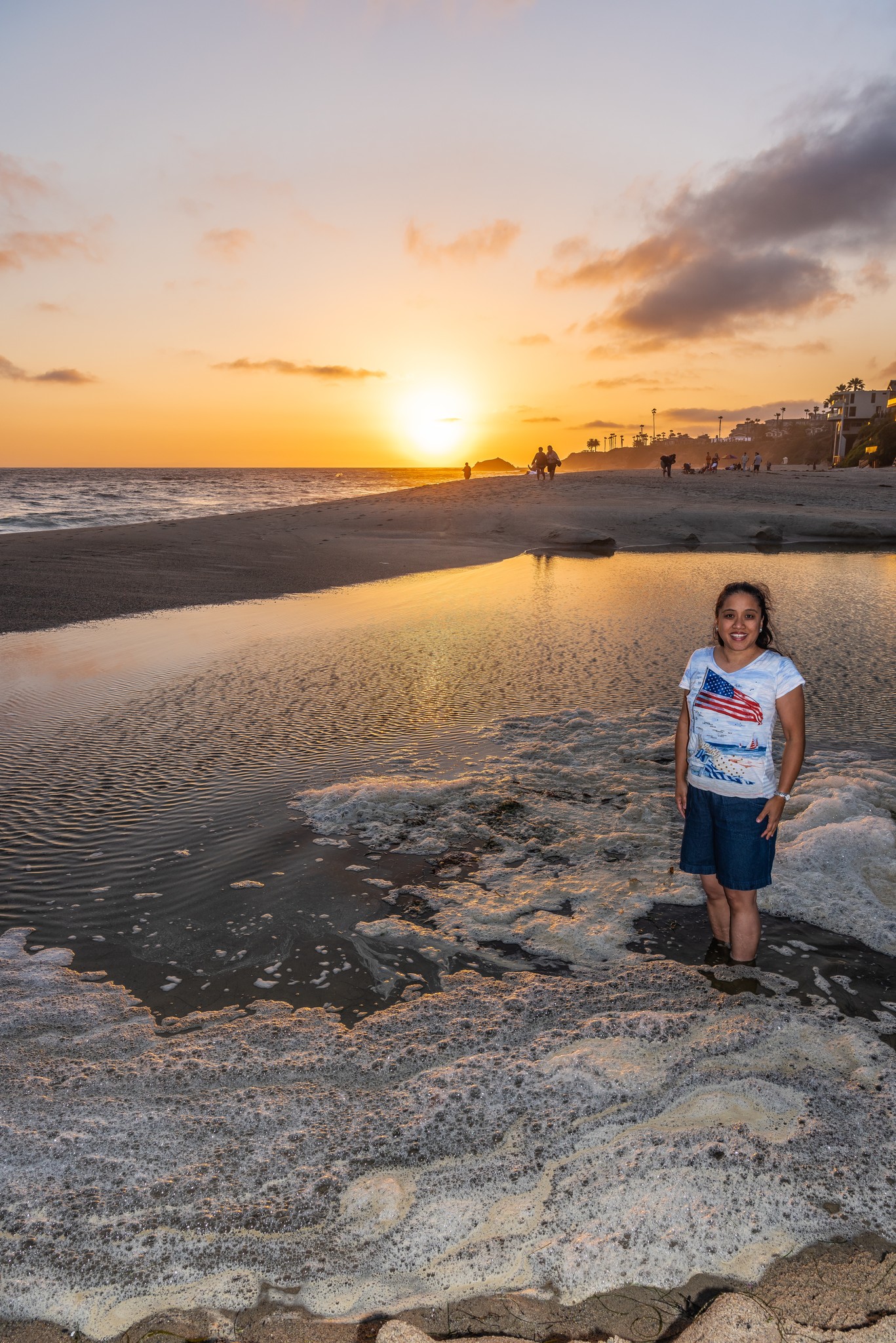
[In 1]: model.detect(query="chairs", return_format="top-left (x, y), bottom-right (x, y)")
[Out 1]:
top-left (696, 466), bottom-right (708, 474)
top-left (781, 457), bottom-right (788, 465)
top-left (708, 463), bottom-right (718, 473)
top-left (680, 463), bottom-right (695, 474)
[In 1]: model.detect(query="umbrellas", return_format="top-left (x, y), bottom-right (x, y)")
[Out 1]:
top-left (719, 454), bottom-right (738, 467)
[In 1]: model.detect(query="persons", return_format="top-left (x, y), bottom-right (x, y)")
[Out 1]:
top-left (660, 454), bottom-right (676, 478)
top-left (712, 453), bottom-right (720, 473)
top-left (706, 452), bottom-right (711, 470)
top-left (806, 461), bottom-right (816, 472)
top-left (674, 581), bottom-right (806, 967)
top-left (546, 446), bottom-right (556, 480)
top-left (729, 461), bottom-right (741, 471)
top-left (463, 463), bottom-right (472, 480)
top-left (528, 461), bottom-right (543, 473)
top-left (752, 452), bottom-right (763, 476)
top-left (766, 461), bottom-right (772, 471)
top-left (741, 452), bottom-right (749, 471)
top-left (532, 447), bottom-right (547, 480)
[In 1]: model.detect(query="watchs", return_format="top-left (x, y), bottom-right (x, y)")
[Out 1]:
top-left (773, 791), bottom-right (791, 801)
